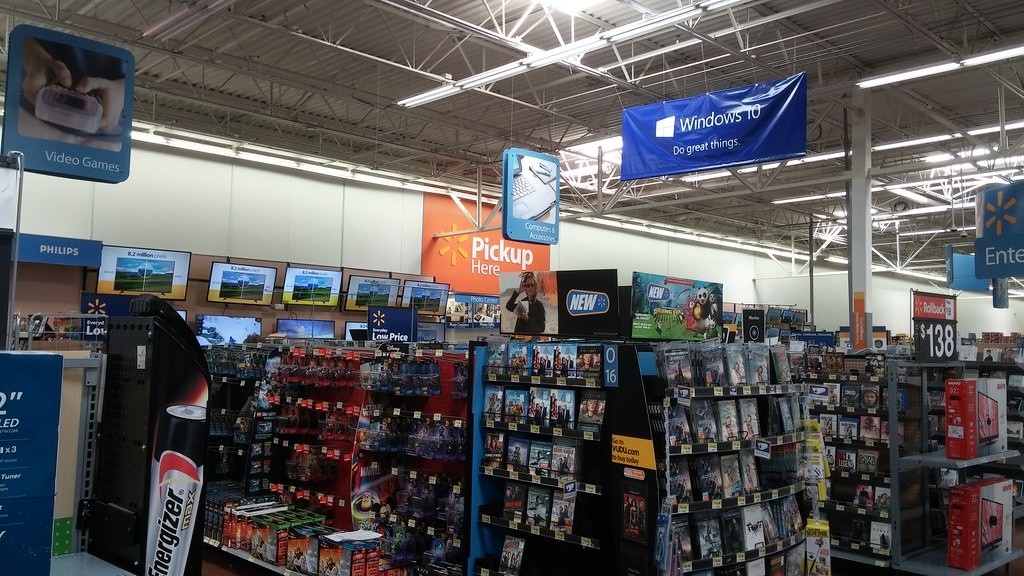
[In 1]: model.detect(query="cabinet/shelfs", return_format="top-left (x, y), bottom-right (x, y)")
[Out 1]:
top-left (179, 341), bottom-right (624, 576)
top-left (616, 332), bottom-right (1024, 576)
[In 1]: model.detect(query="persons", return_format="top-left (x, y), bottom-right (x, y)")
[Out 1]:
top-left (743, 414), bottom-right (754, 440)
top-left (725, 466), bottom-right (733, 487)
top-left (745, 464), bottom-right (753, 490)
top-left (730, 363), bottom-right (742, 387)
top-left (500, 550), bottom-right (521, 574)
top-left (488, 346), bottom-right (551, 376)
top-left (624, 497), bottom-right (644, 529)
top-left (506, 272), bottom-right (546, 334)
top-left (755, 366), bottom-right (763, 385)
top-left (489, 390), bottom-right (605, 429)
top-left (1006, 349), bottom-right (1015, 363)
top-left (674, 360), bottom-right (684, 383)
top-left (721, 415), bottom-right (733, 441)
top-left (984, 350), bottom-right (993, 361)
top-left (824, 385), bottom-right (902, 509)
top-left (485, 435), bottom-right (571, 530)
top-left (577, 354), bottom-right (600, 376)
top-left (553, 346), bottom-right (574, 377)
top-left (674, 420), bottom-right (720, 563)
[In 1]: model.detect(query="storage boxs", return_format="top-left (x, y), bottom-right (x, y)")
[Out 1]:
top-left (957, 344), bottom-right (977, 361)
top-left (944, 377), bottom-right (1007, 461)
top-left (982, 347), bottom-right (1001, 362)
top-left (945, 476), bottom-right (1014, 570)
top-left (236, 501), bottom-right (412, 576)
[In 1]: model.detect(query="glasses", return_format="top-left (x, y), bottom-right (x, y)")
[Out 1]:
top-left (524, 283), bottom-right (536, 288)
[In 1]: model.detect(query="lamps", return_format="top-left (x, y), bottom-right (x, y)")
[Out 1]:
top-left (392, 0), bottom-right (1024, 236)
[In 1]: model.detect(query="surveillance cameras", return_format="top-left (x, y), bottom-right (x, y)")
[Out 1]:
top-left (960, 230), bottom-right (967, 238)
top-left (950, 225), bottom-right (957, 233)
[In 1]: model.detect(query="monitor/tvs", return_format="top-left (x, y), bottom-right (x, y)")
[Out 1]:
top-left (276, 318), bottom-right (335, 340)
top-left (417, 321), bottom-right (445, 344)
top-left (280, 267), bottom-right (343, 307)
top-left (95, 244), bottom-right (192, 301)
top-left (195, 314), bottom-right (262, 347)
top-left (344, 320), bottom-right (368, 341)
top-left (205, 261), bottom-right (278, 306)
top-left (400, 280), bottom-right (450, 316)
top-left (344, 275), bottom-right (401, 312)
